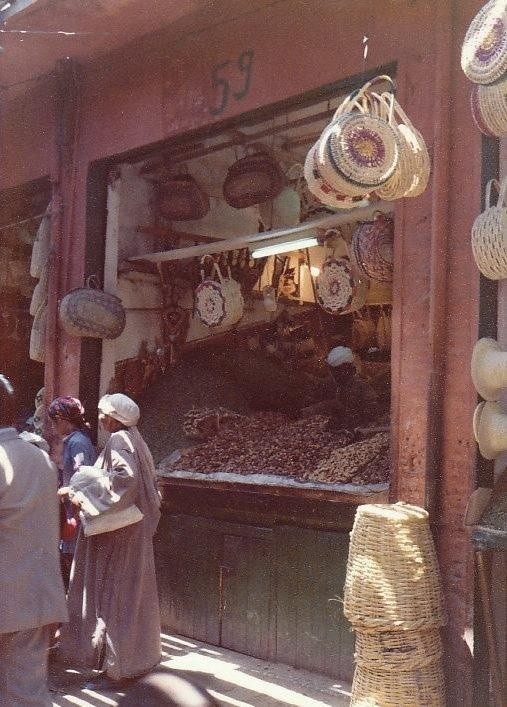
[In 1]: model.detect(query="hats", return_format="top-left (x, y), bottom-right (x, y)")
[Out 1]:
top-left (326, 346), bottom-right (355, 368)
top-left (471, 336), bottom-right (507, 401)
top-left (473, 400), bottom-right (507, 461)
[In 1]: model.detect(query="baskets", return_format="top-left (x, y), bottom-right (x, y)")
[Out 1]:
top-left (342, 501), bottom-right (449, 707)
top-left (460, 0), bottom-right (507, 85)
top-left (469, 75), bottom-right (507, 140)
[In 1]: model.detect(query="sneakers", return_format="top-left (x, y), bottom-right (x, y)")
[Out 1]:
top-left (82, 670), bottom-right (132, 690)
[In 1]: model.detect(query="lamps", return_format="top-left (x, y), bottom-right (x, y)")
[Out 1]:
top-left (249, 227), bottom-right (321, 260)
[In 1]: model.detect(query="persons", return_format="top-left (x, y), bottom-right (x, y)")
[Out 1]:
top-left (45, 394), bottom-right (100, 595)
top-left (51, 393), bottom-right (165, 686)
top-left (0, 373), bottom-right (75, 706)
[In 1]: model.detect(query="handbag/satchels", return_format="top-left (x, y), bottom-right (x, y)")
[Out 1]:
top-left (58, 273), bottom-right (126, 340)
top-left (158, 73), bottom-right (431, 228)
top-left (158, 163), bottom-right (210, 221)
top-left (30, 260), bottom-right (48, 316)
top-left (122, 261), bottom-right (165, 310)
top-left (304, 76), bottom-right (431, 210)
top-left (29, 217), bottom-right (51, 277)
top-left (350, 210), bottom-right (395, 283)
top-left (471, 177), bottom-right (507, 282)
top-left (315, 228), bottom-right (371, 317)
top-left (193, 252), bottom-right (245, 328)
top-left (223, 144), bottom-right (283, 210)
top-left (29, 301), bottom-right (47, 364)
top-left (79, 435), bottom-right (145, 538)
